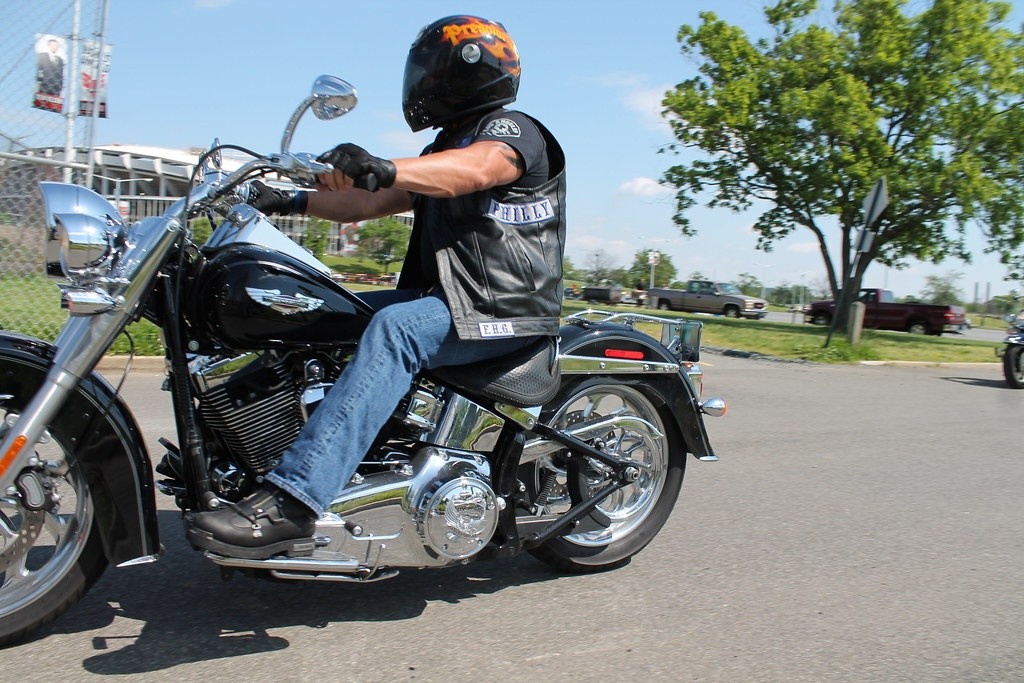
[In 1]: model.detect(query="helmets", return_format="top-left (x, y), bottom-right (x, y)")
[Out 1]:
top-left (401, 15), bottom-right (521, 132)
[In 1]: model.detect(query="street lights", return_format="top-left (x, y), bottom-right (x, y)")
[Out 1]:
top-left (637, 234), bottom-right (672, 288)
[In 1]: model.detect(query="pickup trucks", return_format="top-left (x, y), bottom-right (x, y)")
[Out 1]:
top-left (647, 279), bottom-right (771, 319)
top-left (800, 288), bottom-right (970, 337)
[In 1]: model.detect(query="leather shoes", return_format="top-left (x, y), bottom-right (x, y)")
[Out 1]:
top-left (187, 483), bottom-right (315, 560)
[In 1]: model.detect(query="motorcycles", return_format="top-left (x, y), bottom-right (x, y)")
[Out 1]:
top-left (0, 75), bottom-right (726, 645)
top-left (994, 290), bottom-right (1024, 388)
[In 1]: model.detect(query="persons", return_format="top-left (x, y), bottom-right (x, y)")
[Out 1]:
top-left (181, 14), bottom-right (567, 561)
top-left (36, 39), bottom-right (64, 96)
top-left (636, 278), bottom-right (646, 305)
top-left (662, 283), bottom-right (669, 289)
